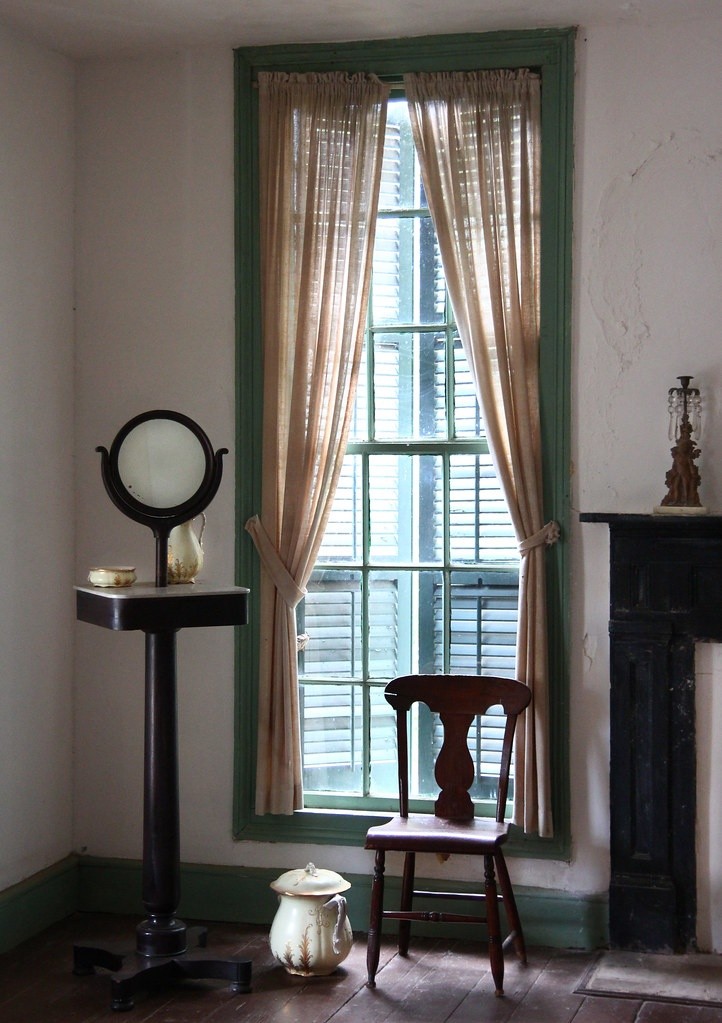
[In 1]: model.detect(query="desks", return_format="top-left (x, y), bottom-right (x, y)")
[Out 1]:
top-left (70, 578), bottom-right (252, 1013)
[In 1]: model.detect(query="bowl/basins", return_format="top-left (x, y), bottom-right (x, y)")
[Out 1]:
top-left (88, 565), bottom-right (137, 588)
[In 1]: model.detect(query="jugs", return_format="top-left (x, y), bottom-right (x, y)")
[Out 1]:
top-left (166, 512), bottom-right (206, 585)
top-left (268, 861), bottom-right (352, 978)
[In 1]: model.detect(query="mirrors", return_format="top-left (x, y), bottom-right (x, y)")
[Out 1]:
top-left (94, 411), bottom-right (228, 586)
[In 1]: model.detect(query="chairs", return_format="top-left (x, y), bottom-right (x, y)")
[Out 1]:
top-left (363, 675), bottom-right (533, 998)
